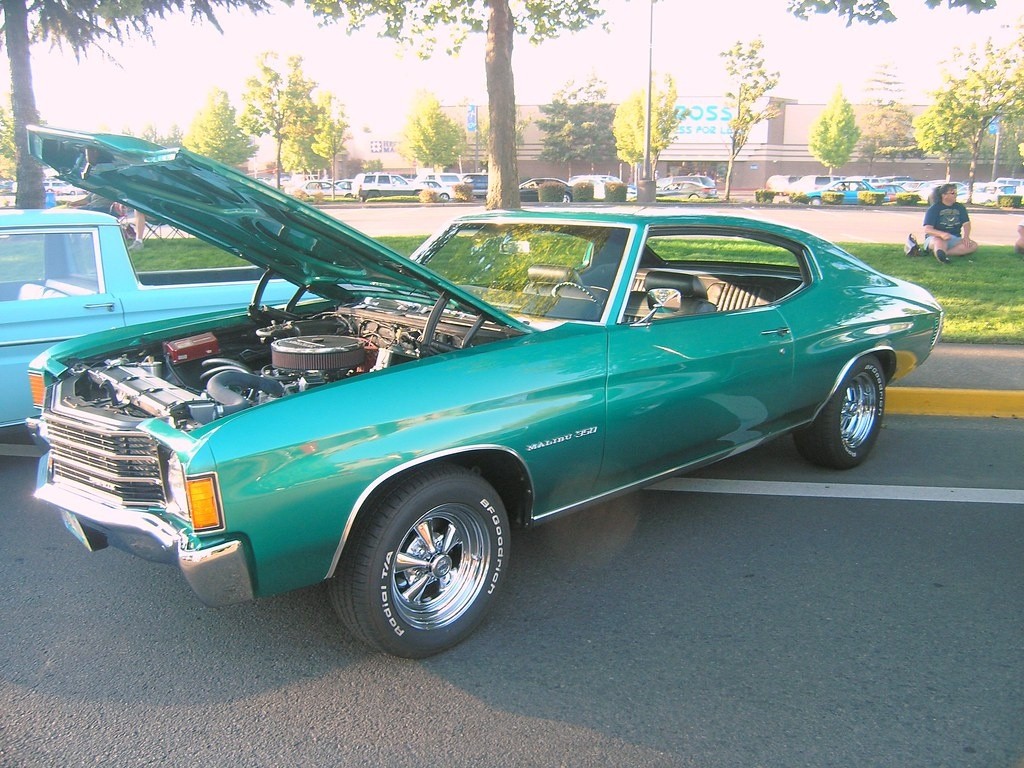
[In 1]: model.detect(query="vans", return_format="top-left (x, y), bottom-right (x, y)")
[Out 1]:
top-left (42, 180), bottom-right (79, 195)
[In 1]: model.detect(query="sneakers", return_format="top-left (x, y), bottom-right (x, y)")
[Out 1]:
top-left (128, 240), bottom-right (146, 252)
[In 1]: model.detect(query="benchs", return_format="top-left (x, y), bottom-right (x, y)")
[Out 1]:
top-left (598, 267), bottom-right (802, 310)
top-left (19, 283), bottom-right (69, 299)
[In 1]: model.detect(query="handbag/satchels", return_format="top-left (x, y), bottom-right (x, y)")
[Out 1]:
top-left (903, 233), bottom-right (921, 256)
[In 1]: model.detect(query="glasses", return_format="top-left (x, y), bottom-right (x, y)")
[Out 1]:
top-left (941, 190), bottom-right (958, 195)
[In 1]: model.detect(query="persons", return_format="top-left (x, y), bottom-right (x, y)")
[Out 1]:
top-left (1016, 220), bottom-right (1024, 251)
top-left (923, 183), bottom-right (976, 262)
top-left (66, 191), bottom-right (123, 219)
top-left (127, 209), bottom-right (145, 250)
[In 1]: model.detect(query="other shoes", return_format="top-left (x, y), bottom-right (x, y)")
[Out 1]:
top-left (933, 248), bottom-right (951, 265)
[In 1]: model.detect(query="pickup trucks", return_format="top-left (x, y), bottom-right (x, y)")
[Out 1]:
top-left (0, 209), bottom-right (317, 427)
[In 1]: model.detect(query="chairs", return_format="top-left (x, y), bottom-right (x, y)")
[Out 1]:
top-left (67, 199), bottom-right (131, 240)
top-left (523, 264), bottom-right (584, 298)
top-left (623, 271), bottom-right (716, 323)
top-left (143, 214), bottom-right (186, 241)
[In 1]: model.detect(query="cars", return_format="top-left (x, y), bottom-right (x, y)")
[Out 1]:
top-left (281, 171), bottom-right (717, 202)
top-left (26, 124), bottom-right (945, 659)
top-left (767, 175), bottom-right (1024, 207)
